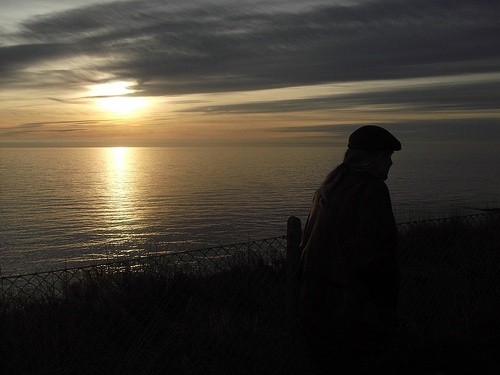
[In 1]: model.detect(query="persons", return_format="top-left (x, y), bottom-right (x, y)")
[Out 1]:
top-left (294, 124), bottom-right (403, 374)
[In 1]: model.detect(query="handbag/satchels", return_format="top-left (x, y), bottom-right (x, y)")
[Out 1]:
top-left (289, 169), bottom-right (394, 353)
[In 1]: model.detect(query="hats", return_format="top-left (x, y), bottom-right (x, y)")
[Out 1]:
top-left (347, 125), bottom-right (402, 152)
